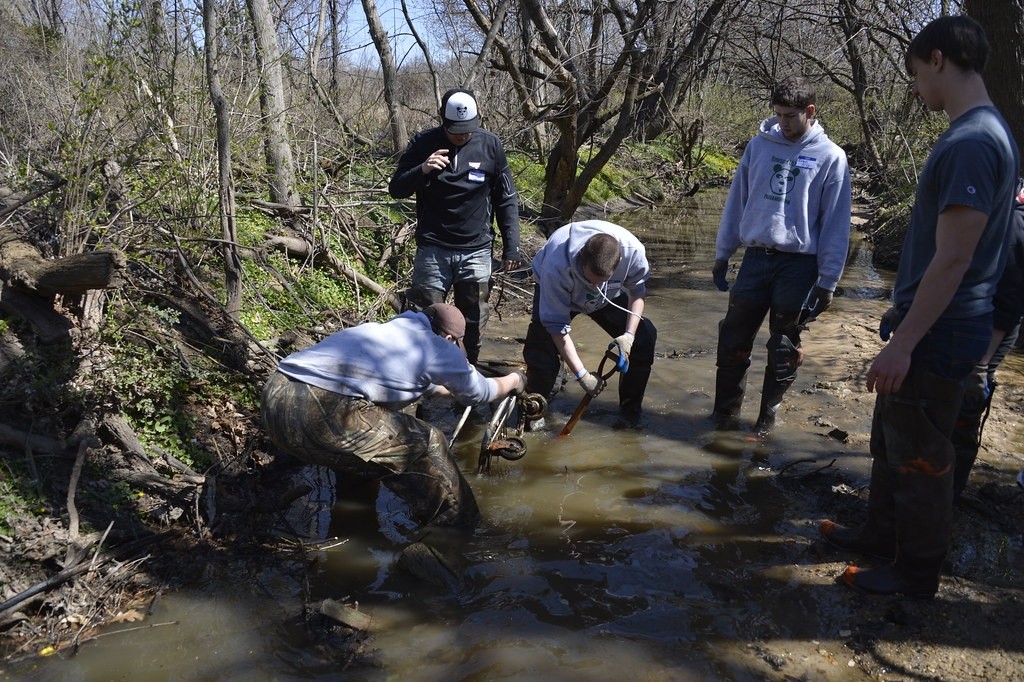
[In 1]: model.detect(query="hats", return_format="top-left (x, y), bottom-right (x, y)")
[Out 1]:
top-left (422, 303), bottom-right (467, 355)
top-left (441, 88), bottom-right (480, 135)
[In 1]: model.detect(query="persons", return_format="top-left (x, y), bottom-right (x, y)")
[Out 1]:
top-left (387, 88), bottom-right (521, 372)
top-left (519, 218), bottom-right (658, 431)
top-left (880, 134), bottom-right (1024, 519)
top-left (816, 16), bottom-right (1016, 599)
top-left (691, 79), bottom-right (852, 442)
top-left (258, 302), bottom-right (527, 544)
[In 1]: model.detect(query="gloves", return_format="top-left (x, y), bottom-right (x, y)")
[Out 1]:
top-left (608, 331), bottom-right (635, 374)
top-left (963, 362), bottom-right (991, 405)
top-left (808, 288), bottom-right (834, 317)
top-left (713, 260), bottom-right (729, 292)
top-left (880, 306), bottom-right (901, 341)
top-left (576, 367), bottom-right (608, 399)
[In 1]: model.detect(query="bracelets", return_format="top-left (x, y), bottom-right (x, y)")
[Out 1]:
top-left (576, 368), bottom-right (587, 378)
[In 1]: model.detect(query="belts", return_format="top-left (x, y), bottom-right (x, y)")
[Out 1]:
top-left (754, 247), bottom-right (816, 258)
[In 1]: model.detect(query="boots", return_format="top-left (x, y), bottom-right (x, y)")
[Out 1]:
top-left (818, 401), bottom-right (896, 563)
top-left (840, 371), bottom-right (966, 601)
top-left (951, 422), bottom-right (980, 501)
top-left (407, 289), bottom-right (448, 309)
top-left (710, 295), bottom-right (770, 418)
top-left (751, 303), bottom-right (804, 431)
top-left (523, 323), bottom-right (560, 398)
top-left (453, 281), bottom-right (488, 367)
top-left (619, 366), bottom-right (652, 414)
